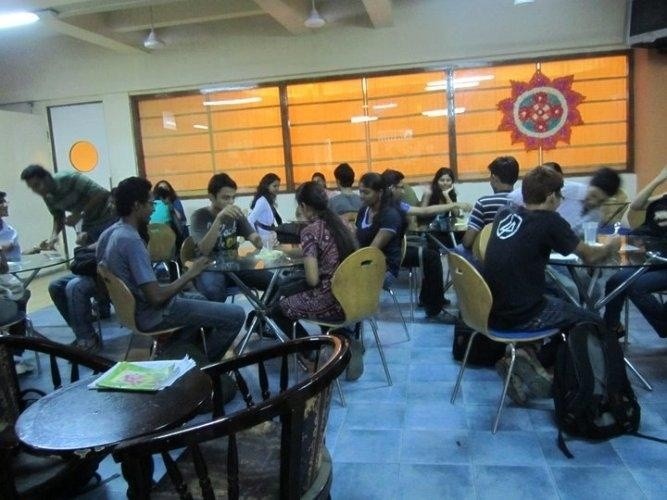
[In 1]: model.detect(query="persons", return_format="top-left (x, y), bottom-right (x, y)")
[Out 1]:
top-left (310, 172), bottom-right (327, 193)
top-left (185, 173), bottom-right (283, 339)
top-left (0, 241), bottom-right (36, 373)
top-left (483, 165), bottom-right (623, 408)
top-left (418, 167), bottom-right (467, 254)
top-left (601, 161), bottom-right (667, 340)
top-left (462, 155), bottom-right (521, 252)
top-left (543, 162), bottom-right (564, 174)
top-left (154, 179), bottom-right (189, 227)
top-left (326, 163), bottom-right (366, 244)
top-left (0, 191), bottom-right (21, 274)
top-left (381, 169), bottom-right (475, 325)
top-left (352, 172), bottom-right (409, 290)
top-left (145, 187), bottom-right (189, 289)
top-left (94, 176), bottom-right (246, 365)
top-left (48, 186), bottom-right (120, 355)
top-left (247, 172), bottom-right (278, 245)
top-left (269, 181), bottom-right (365, 383)
top-left (19, 163), bottom-right (114, 322)
top-left (509, 167), bottom-right (623, 231)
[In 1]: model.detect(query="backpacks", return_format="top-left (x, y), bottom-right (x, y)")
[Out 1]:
top-left (553, 318), bottom-right (667, 459)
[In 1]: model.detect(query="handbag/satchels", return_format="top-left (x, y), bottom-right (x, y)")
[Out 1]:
top-left (69, 249), bottom-right (97, 275)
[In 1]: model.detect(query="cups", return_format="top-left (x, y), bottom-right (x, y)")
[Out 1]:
top-left (583, 222), bottom-right (598, 245)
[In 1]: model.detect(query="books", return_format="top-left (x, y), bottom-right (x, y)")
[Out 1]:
top-left (86, 354), bottom-right (197, 393)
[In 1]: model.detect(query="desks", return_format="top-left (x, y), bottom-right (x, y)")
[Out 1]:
top-left (407, 215), bottom-right (471, 286)
top-left (14, 359), bottom-right (213, 500)
top-left (184, 244), bottom-right (310, 376)
top-left (0, 250), bottom-right (70, 287)
top-left (548, 235), bottom-right (667, 390)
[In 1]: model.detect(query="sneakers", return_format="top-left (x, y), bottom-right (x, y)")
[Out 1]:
top-left (507, 342), bottom-right (554, 399)
top-left (426, 309), bottom-right (457, 324)
top-left (495, 358), bottom-right (528, 405)
top-left (74, 338), bottom-right (98, 355)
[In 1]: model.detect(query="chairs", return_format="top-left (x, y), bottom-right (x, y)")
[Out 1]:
top-left (181, 234), bottom-right (199, 272)
top-left (402, 266), bottom-right (417, 312)
top-left (113, 335), bottom-right (350, 500)
top-left (292, 247), bottom-right (392, 404)
top-left (98, 262), bottom-right (186, 364)
top-left (0, 334), bottom-right (131, 500)
top-left (446, 251), bottom-right (561, 434)
top-left (385, 231), bottom-right (409, 346)
top-left (143, 222), bottom-right (179, 280)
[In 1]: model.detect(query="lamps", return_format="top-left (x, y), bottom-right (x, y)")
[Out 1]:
top-left (145, 0), bottom-right (162, 49)
top-left (305, 0), bottom-right (326, 27)
top-left (34, 0), bottom-right (59, 20)
top-left (514, 0), bottom-right (534, 7)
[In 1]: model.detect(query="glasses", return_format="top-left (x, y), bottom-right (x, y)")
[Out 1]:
top-left (139, 199), bottom-right (157, 205)
top-left (394, 185), bottom-right (404, 189)
top-left (557, 191), bottom-right (564, 201)
